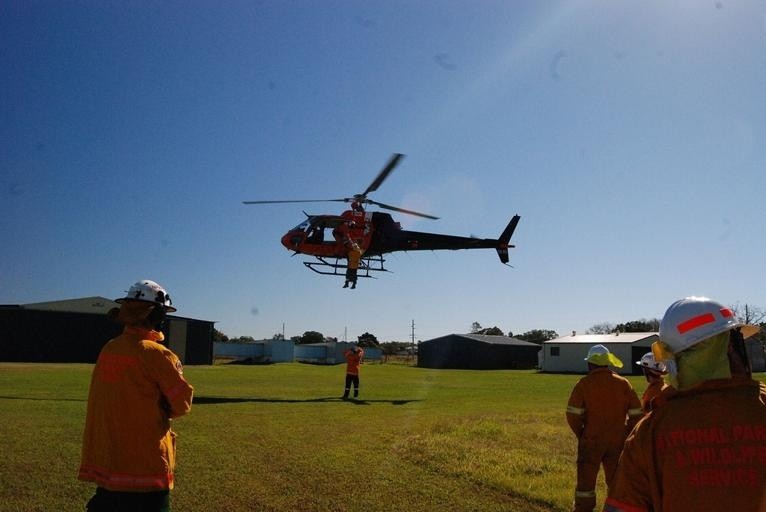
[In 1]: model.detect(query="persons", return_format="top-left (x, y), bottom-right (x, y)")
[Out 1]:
top-left (635, 350), bottom-right (678, 414)
top-left (599, 294), bottom-right (765, 511)
top-left (339, 345), bottom-right (365, 403)
top-left (342, 238), bottom-right (365, 289)
top-left (77, 280), bottom-right (196, 512)
top-left (563, 344), bottom-right (646, 511)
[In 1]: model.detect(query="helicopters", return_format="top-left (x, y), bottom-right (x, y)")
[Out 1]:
top-left (242, 153), bottom-right (520, 278)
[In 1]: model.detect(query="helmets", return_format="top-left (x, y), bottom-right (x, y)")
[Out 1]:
top-left (635, 352), bottom-right (667, 372)
top-left (658, 295), bottom-right (761, 357)
top-left (113, 279), bottom-right (177, 312)
top-left (584, 344), bottom-right (624, 368)
